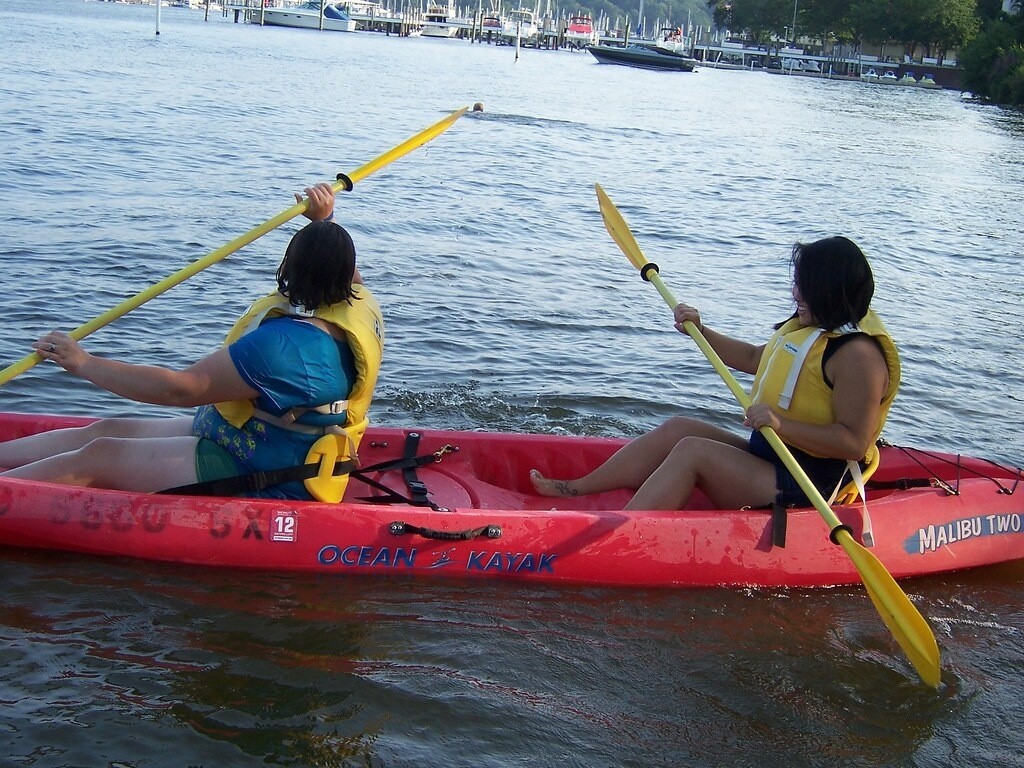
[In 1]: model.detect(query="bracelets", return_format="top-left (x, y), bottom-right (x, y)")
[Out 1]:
top-left (312, 210), bottom-right (334, 223)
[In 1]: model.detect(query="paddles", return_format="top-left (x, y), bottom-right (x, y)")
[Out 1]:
top-left (594, 181), bottom-right (945, 693)
top-left (1, 98), bottom-right (471, 390)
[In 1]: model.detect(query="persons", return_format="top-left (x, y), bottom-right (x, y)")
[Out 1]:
top-left (531, 234), bottom-right (902, 511)
top-left (1, 182), bottom-right (384, 504)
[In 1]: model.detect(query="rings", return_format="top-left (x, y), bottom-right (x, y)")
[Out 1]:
top-left (48, 343), bottom-right (55, 353)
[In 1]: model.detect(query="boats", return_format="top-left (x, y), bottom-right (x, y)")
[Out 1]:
top-left (1, 413), bottom-right (1024, 588)
top-left (86, 0), bottom-right (943, 93)
